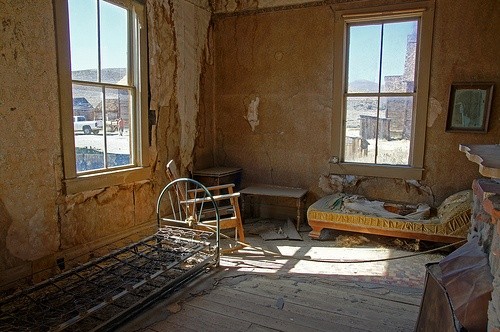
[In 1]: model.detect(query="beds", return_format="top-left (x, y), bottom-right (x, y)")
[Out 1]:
top-left (1, 177), bottom-right (221, 331)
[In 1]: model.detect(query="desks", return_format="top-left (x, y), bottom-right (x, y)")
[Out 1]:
top-left (192, 165), bottom-right (243, 207)
top-left (237, 184), bottom-right (308, 233)
top-left (460, 144), bottom-right (499, 281)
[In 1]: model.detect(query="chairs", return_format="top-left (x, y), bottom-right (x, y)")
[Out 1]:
top-left (166, 161), bottom-right (251, 254)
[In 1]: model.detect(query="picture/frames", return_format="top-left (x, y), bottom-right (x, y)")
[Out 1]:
top-left (444, 82), bottom-right (496, 135)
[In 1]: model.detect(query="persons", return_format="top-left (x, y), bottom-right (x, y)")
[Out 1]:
top-left (118, 118), bottom-right (124, 136)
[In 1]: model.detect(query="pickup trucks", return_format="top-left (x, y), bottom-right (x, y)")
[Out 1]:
top-left (73, 115), bottom-right (102, 135)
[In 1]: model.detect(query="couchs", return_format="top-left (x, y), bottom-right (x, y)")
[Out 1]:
top-left (306, 189), bottom-right (473, 244)
